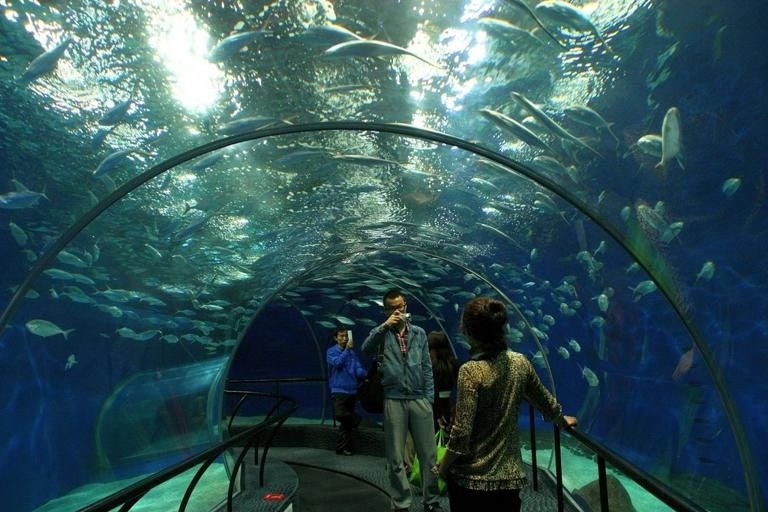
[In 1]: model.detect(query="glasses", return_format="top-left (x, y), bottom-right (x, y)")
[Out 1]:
top-left (385, 302), bottom-right (407, 315)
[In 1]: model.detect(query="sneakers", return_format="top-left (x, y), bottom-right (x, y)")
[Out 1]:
top-left (424, 502), bottom-right (447, 512)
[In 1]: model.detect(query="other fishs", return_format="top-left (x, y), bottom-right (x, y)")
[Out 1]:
top-left (0, 91), bottom-right (615, 388)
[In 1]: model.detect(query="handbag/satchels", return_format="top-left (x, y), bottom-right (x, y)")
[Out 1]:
top-left (410, 428), bottom-right (448, 494)
top-left (357, 376), bottom-right (384, 414)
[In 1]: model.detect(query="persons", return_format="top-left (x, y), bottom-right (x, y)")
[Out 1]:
top-left (325, 327), bottom-right (369, 456)
top-left (361, 290), bottom-right (445, 512)
top-left (430, 296), bottom-right (577, 512)
top-left (428, 331), bottom-right (458, 447)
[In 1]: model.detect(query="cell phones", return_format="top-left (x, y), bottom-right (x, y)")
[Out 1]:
top-left (347, 330), bottom-right (354, 342)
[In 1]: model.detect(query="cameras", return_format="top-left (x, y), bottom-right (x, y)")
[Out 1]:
top-left (400, 313), bottom-right (412, 321)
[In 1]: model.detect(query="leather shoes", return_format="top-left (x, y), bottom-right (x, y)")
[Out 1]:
top-left (336, 448), bottom-right (352, 456)
